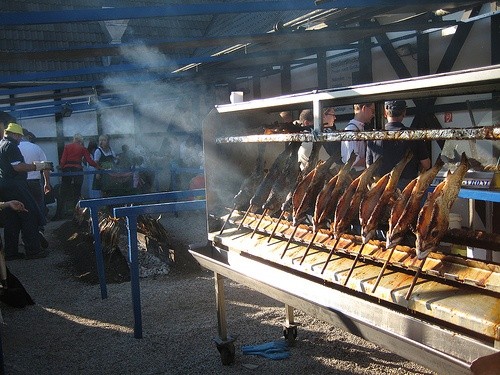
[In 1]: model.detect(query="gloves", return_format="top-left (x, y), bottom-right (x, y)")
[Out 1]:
top-left (33, 161), bottom-right (51, 170)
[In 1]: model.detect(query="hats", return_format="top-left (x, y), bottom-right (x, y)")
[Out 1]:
top-left (5, 123), bottom-right (25, 137)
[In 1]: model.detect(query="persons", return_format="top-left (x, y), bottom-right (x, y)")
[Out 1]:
top-left (83, 142), bottom-right (97, 169)
top-left (118, 145), bottom-right (136, 168)
top-left (0, 123), bottom-right (52, 260)
top-left (378, 100), bottom-right (430, 180)
top-left (340, 101), bottom-right (375, 171)
top-left (298, 109), bottom-right (314, 171)
top-left (321, 104), bottom-right (341, 161)
top-left (92, 135), bottom-right (116, 197)
top-left (279, 111), bottom-right (293, 125)
top-left (18, 129), bottom-right (52, 249)
top-left (60, 134), bottom-right (103, 208)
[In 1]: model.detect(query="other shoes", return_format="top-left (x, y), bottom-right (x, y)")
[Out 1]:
top-left (25, 253), bottom-right (41, 260)
top-left (37, 230), bottom-right (49, 250)
top-left (5, 252), bottom-right (24, 261)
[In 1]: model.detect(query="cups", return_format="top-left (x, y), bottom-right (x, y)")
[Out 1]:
top-left (230, 91), bottom-right (243, 103)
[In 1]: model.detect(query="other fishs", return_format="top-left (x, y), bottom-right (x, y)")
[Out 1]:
top-left (232, 136), bottom-right (482, 261)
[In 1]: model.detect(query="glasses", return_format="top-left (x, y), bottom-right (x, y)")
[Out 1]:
top-left (322, 112), bottom-right (335, 118)
